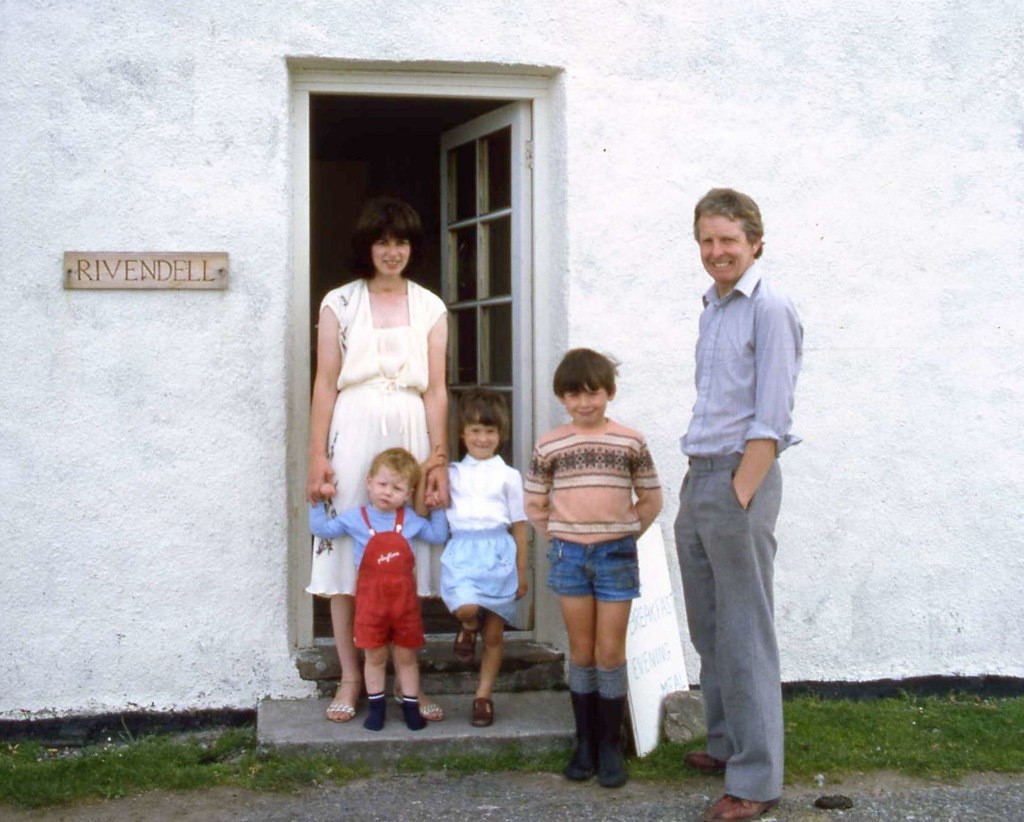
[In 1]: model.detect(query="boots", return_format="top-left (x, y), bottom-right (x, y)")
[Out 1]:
top-left (597, 692), bottom-right (630, 788)
top-left (565, 691), bottom-right (596, 781)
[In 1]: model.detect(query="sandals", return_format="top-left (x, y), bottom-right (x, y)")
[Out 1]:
top-left (327, 672), bottom-right (362, 723)
top-left (392, 687), bottom-right (444, 722)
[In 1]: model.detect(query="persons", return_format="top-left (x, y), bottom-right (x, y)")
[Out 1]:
top-left (410, 377), bottom-right (532, 733)
top-left (672, 186), bottom-right (807, 822)
top-left (303, 209), bottom-right (456, 729)
top-left (307, 440), bottom-right (450, 738)
top-left (520, 346), bottom-right (665, 793)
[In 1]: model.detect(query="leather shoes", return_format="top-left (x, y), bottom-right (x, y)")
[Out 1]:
top-left (472, 697), bottom-right (494, 726)
top-left (453, 620), bottom-right (481, 664)
top-left (688, 751), bottom-right (726, 771)
top-left (705, 793), bottom-right (781, 822)
